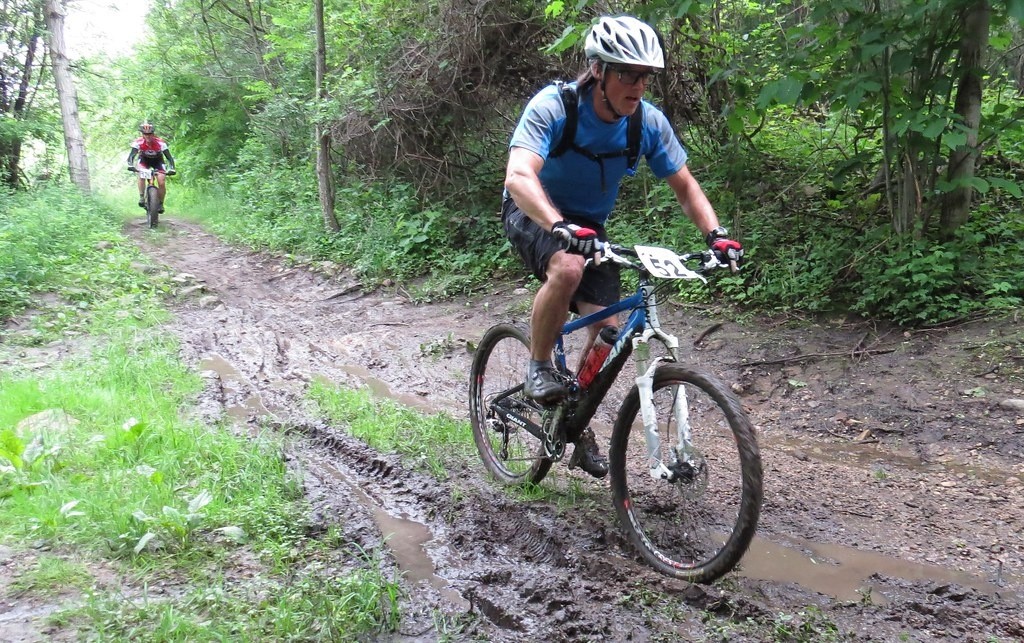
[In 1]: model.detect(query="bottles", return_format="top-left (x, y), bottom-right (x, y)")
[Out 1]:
top-left (578, 325), bottom-right (619, 387)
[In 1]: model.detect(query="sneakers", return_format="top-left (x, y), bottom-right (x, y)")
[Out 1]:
top-left (574, 427), bottom-right (609, 479)
top-left (523, 369), bottom-right (564, 403)
top-left (158, 205), bottom-right (164, 214)
top-left (139, 199), bottom-right (145, 207)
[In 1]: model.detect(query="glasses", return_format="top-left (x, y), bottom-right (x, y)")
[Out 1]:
top-left (600, 61), bottom-right (659, 86)
top-left (144, 133), bottom-right (152, 136)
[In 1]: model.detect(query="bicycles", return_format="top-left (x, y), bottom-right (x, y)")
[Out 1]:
top-left (465, 231), bottom-right (767, 583)
top-left (127, 167), bottom-right (179, 230)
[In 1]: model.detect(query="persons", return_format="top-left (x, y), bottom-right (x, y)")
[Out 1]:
top-left (128, 124), bottom-right (176, 215)
top-left (500, 16), bottom-right (744, 477)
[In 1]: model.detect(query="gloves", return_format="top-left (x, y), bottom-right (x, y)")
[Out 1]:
top-left (549, 221), bottom-right (602, 267)
top-left (705, 227), bottom-right (745, 273)
top-left (167, 170), bottom-right (176, 177)
top-left (127, 167), bottom-right (137, 173)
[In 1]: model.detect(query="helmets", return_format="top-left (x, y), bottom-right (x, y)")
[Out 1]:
top-left (584, 15), bottom-right (665, 68)
top-left (139, 124), bottom-right (155, 134)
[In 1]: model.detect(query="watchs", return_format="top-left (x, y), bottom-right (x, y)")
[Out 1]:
top-left (706, 226), bottom-right (728, 245)
